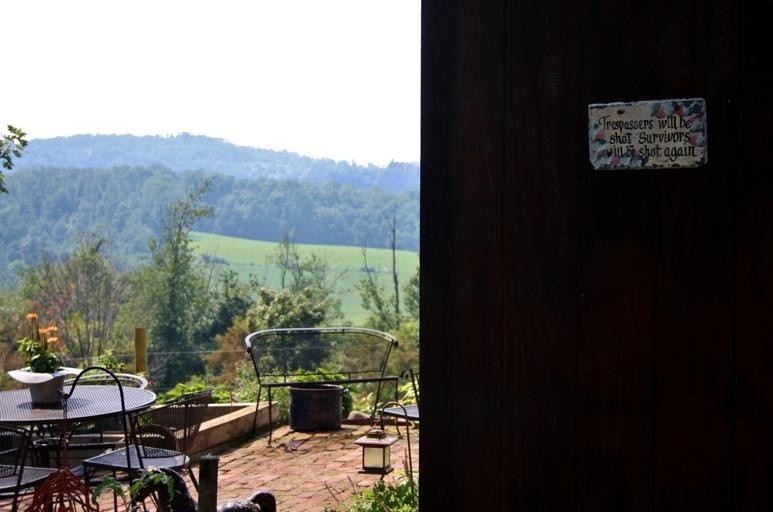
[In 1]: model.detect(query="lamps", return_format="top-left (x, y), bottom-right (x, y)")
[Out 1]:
top-left (352, 402), bottom-right (419, 511)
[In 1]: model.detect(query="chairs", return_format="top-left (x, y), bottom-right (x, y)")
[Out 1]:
top-left (1, 373), bottom-right (214, 512)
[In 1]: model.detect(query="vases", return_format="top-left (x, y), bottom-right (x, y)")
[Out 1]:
top-left (9, 368), bottom-right (83, 404)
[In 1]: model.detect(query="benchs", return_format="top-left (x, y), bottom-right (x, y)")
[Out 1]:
top-left (244, 326), bottom-right (400, 450)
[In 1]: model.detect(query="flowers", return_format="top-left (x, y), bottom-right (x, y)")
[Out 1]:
top-left (16, 314), bottom-right (63, 372)
